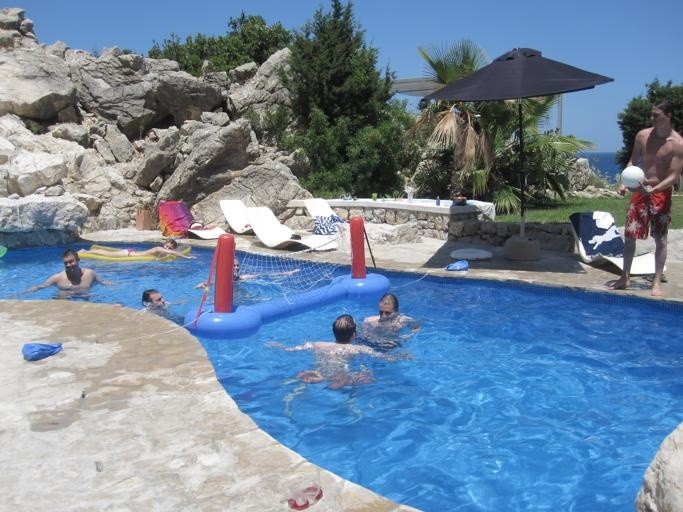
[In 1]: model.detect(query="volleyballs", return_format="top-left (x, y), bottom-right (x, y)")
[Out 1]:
top-left (622, 166), bottom-right (644, 188)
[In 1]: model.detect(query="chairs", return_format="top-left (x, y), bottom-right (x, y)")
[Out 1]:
top-left (568, 211), bottom-right (667, 277)
top-left (158, 197), bottom-right (352, 250)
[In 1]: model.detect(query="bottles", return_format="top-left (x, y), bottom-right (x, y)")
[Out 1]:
top-left (436, 195), bottom-right (441, 206)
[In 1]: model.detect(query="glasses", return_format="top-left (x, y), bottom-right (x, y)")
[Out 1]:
top-left (287, 484), bottom-right (324, 510)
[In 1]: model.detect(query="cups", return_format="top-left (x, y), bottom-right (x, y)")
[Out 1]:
top-left (372, 192), bottom-right (377, 202)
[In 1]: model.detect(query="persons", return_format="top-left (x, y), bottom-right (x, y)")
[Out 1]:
top-left (78, 238), bottom-right (198, 260)
top-left (10, 249), bottom-right (118, 300)
top-left (138, 289), bottom-right (187, 317)
top-left (607, 101), bottom-right (683, 296)
top-left (355, 291), bottom-right (422, 375)
top-left (266, 313), bottom-right (415, 417)
top-left (193, 254), bottom-right (301, 291)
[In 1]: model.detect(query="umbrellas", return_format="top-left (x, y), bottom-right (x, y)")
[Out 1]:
top-left (419, 47), bottom-right (616, 241)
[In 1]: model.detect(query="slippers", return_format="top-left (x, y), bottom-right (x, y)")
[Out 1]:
top-left (642, 273), bottom-right (667, 282)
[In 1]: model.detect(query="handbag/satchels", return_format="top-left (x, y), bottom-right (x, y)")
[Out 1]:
top-left (135, 203), bottom-right (153, 231)
